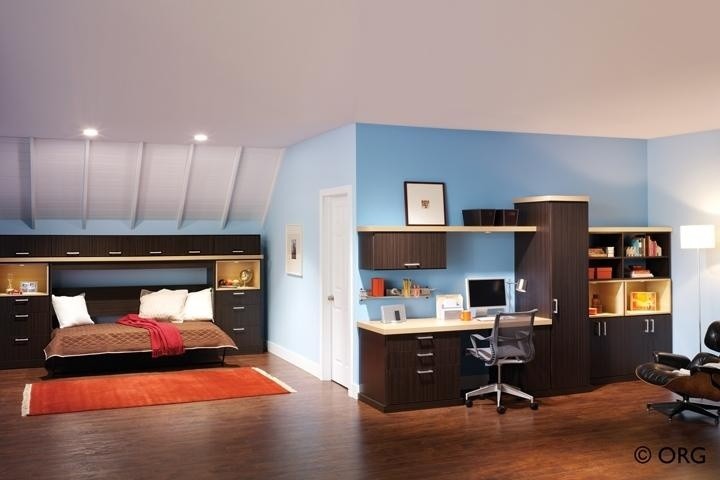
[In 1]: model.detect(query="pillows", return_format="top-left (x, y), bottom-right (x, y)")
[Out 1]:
top-left (51, 292), bottom-right (95, 329)
top-left (138, 288), bottom-right (188, 324)
top-left (183, 287), bottom-right (215, 323)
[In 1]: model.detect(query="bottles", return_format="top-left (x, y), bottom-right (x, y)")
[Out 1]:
top-left (592, 293), bottom-right (602, 313)
top-left (403, 278), bottom-right (411, 296)
top-left (413, 285), bottom-right (421, 297)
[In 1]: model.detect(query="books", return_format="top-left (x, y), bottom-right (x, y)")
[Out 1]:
top-left (588, 235), bottom-right (663, 259)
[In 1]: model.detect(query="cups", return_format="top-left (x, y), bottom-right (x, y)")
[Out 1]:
top-left (371, 278), bottom-right (385, 297)
top-left (459, 310), bottom-right (472, 321)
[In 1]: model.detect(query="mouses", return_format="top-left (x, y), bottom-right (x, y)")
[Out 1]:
top-left (507, 316), bottom-right (514, 320)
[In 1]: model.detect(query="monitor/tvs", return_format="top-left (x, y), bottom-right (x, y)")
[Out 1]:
top-left (465, 277), bottom-right (508, 317)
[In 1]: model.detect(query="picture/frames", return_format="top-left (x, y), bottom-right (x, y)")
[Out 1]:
top-left (629, 291), bottom-right (656, 311)
top-left (404, 181), bottom-right (447, 226)
top-left (284, 224), bottom-right (304, 277)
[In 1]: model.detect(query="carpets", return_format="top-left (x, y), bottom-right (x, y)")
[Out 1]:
top-left (20, 366), bottom-right (296, 418)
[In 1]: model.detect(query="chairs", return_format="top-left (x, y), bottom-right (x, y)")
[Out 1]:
top-left (635, 321), bottom-right (720, 426)
top-left (464, 308), bottom-right (539, 414)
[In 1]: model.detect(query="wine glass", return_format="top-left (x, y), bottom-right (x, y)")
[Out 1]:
top-left (7, 272), bottom-right (14, 288)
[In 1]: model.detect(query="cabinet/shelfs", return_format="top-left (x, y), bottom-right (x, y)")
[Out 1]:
top-left (358, 226), bottom-right (537, 271)
top-left (207, 261), bottom-right (266, 354)
top-left (588, 227), bottom-right (672, 385)
top-left (0, 262), bottom-right (53, 368)
top-left (512, 194), bottom-right (590, 395)
top-left (0, 234), bottom-right (265, 263)
top-left (358, 316), bottom-right (551, 414)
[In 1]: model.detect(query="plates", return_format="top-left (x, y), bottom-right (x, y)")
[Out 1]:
top-left (219, 283), bottom-right (240, 288)
top-left (628, 265), bottom-right (644, 270)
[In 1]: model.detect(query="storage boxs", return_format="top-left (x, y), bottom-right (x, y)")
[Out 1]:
top-left (496, 209), bottom-right (519, 226)
top-left (461, 209), bottom-right (496, 225)
top-left (588, 266), bottom-right (612, 280)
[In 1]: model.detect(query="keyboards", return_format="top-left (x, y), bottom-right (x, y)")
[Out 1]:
top-left (477, 316), bottom-right (503, 322)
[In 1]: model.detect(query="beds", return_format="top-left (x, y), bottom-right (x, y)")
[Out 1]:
top-left (42, 284), bottom-right (239, 378)
